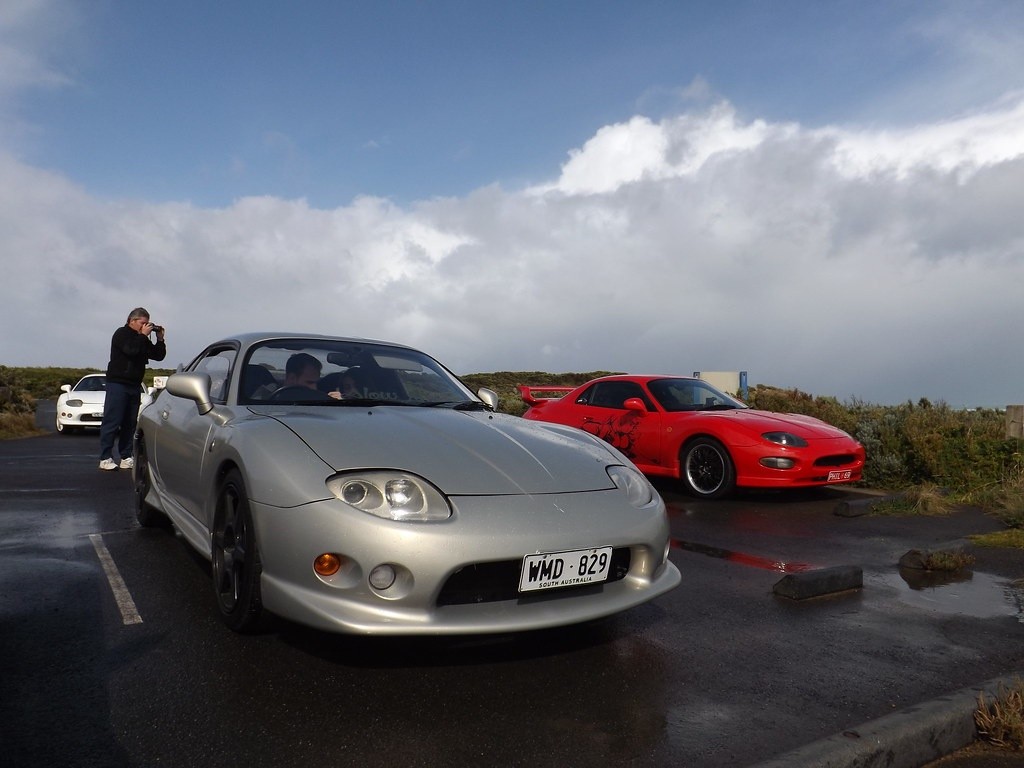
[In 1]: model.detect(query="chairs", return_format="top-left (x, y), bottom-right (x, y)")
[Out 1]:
top-left (318, 373), bottom-right (344, 394)
top-left (241, 364), bottom-right (277, 398)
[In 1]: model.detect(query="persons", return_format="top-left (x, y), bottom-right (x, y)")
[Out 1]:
top-left (97, 307), bottom-right (167, 470)
top-left (251, 352), bottom-right (342, 400)
top-left (336, 367), bottom-right (377, 398)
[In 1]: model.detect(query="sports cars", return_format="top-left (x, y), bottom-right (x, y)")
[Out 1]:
top-left (56, 374), bottom-right (158, 434)
top-left (131, 331), bottom-right (684, 638)
top-left (516, 374), bottom-right (867, 499)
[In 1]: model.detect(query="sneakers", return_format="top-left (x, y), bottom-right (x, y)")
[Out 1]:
top-left (120, 456), bottom-right (135, 469)
top-left (99, 458), bottom-right (118, 470)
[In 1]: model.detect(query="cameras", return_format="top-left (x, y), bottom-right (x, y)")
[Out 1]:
top-left (146, 323), bottom-right (162, 332)
